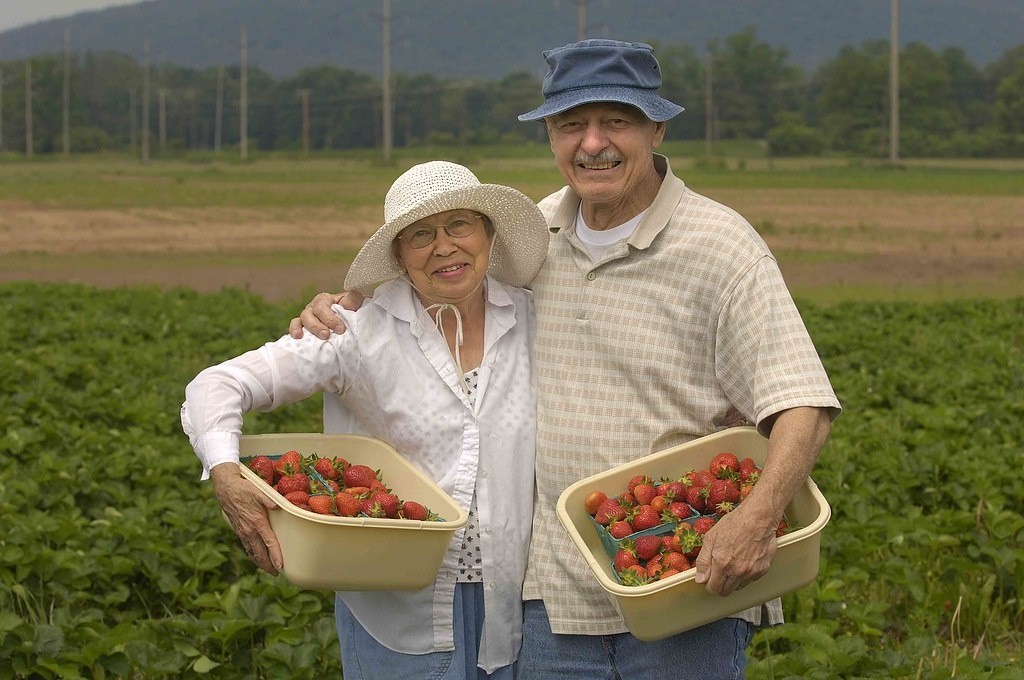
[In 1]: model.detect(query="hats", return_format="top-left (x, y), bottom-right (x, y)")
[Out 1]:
top-left (343, 160), bottom-right (550, 298)
top-left (517, 39), bottom-right (686, 123)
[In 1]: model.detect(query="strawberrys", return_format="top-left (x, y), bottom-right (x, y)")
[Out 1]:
top-left (584, 453), bottom-right (789, 583)
top-left (245, 451), bottom-right (428, 521)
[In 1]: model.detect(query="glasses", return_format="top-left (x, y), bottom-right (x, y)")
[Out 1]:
top-left (398, 216), bottom-right (482, 250)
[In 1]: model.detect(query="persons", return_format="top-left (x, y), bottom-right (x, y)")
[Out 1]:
top-left (181, 160), bottom-right (548, 680)
top-left (290, 39), bottom-right (840, 680)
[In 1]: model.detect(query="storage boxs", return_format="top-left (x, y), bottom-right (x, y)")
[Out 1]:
top-left (222, 433), bottom-right (469, 589)
top-left (555, 426), bottom-right (831, 644)
